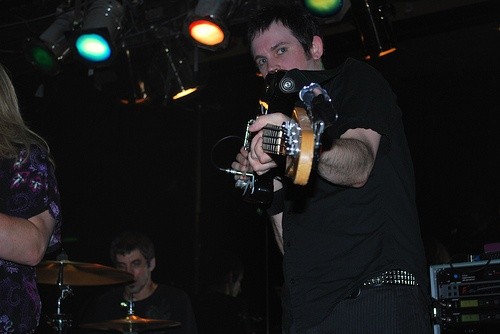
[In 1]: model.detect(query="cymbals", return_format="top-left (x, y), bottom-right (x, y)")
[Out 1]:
top-left (82, 316), bottom-right (182, 329)
top-left (36, 259), bottom-right (134, 284)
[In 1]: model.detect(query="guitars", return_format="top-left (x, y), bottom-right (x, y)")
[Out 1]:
top-left (218, 99), bottom-right (315, 203)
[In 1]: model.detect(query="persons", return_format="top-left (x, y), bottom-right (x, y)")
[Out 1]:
top-left (232, 1), bottom-right (435, 334)
top-left (72, 232), bottom-right (197, 333)
top-left (0, 66), bottom-right (64, 334)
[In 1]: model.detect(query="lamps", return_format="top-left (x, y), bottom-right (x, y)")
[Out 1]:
top-left (27, 0), bottom-right (243, 107)
top-left (295, 0), bottom-right (397, 60)
